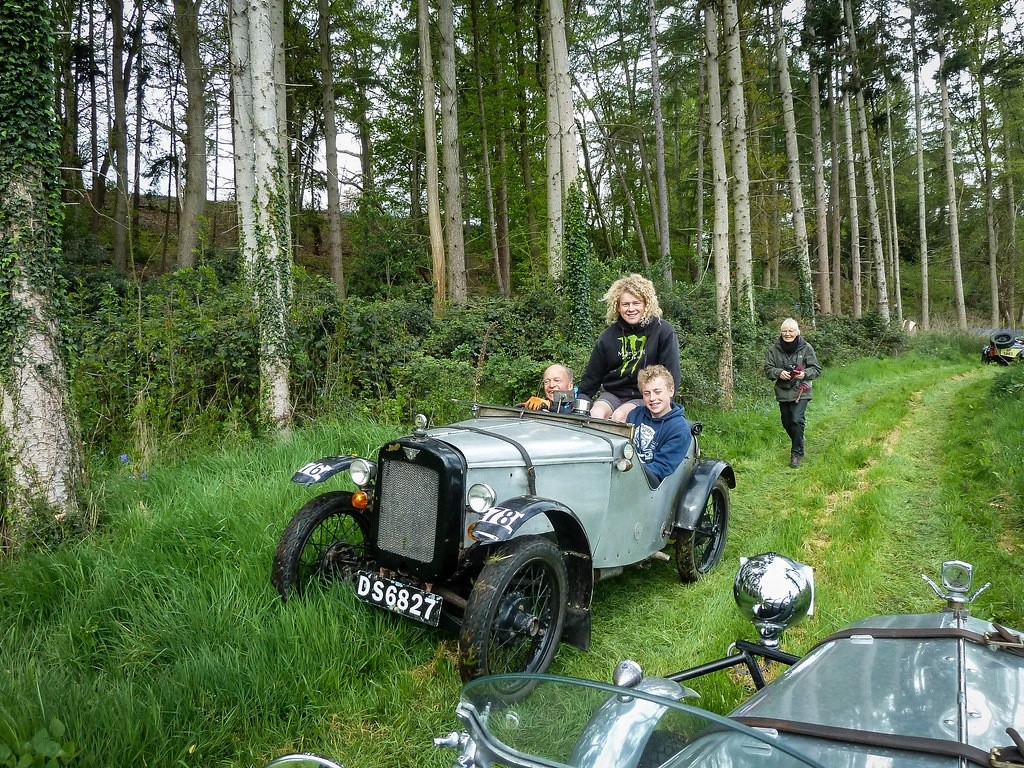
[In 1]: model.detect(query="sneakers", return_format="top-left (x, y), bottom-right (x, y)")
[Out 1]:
top-left (790, 451), bottom-right (802, 468)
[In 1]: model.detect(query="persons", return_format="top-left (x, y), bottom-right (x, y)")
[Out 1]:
top-left (574, 273), bottom-right (681, 423)
top-left (525, 363), bottom-right (575, 411)
top-left (764, 318), bottom-right (822, 469)
top-left (624, 364), bottom-right (692, 489)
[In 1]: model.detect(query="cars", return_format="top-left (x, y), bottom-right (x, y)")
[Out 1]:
top-left (426, 553), bottom-right (1024, 768)
top-left (268, 391), bottom-right (736, 705)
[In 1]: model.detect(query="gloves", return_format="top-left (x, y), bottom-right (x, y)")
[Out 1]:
top-left (525, 396), bottom-right (551, 413)
